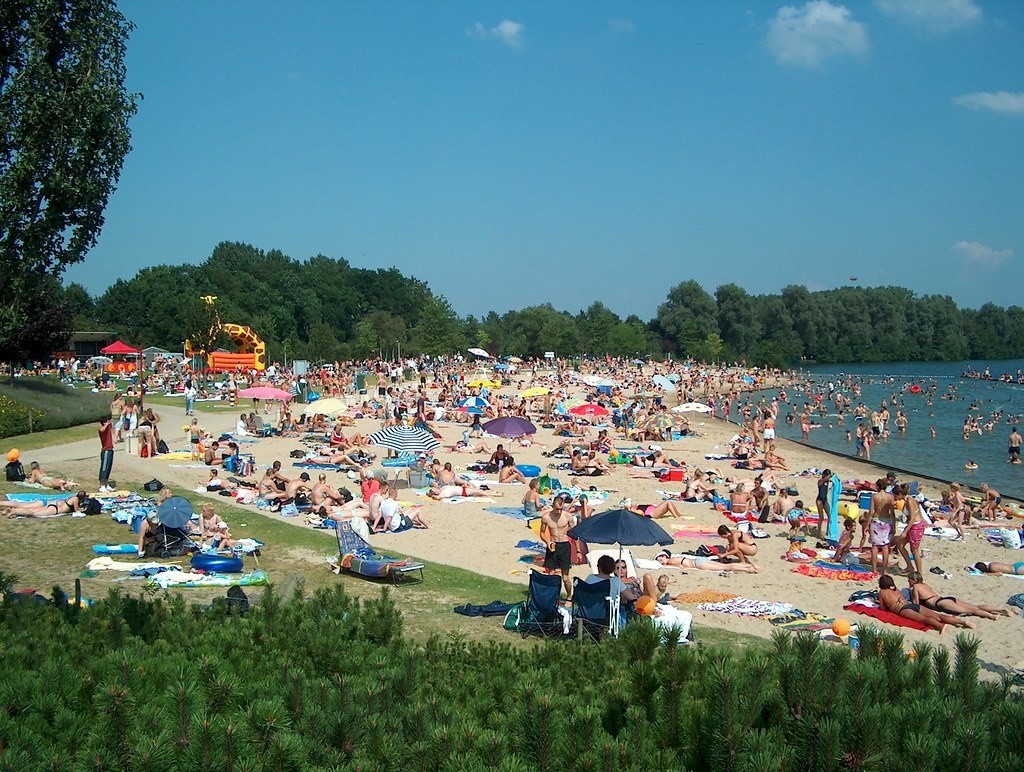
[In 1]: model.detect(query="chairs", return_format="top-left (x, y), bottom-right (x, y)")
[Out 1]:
top-left (517, 549), bottom-right (695, 645)
top-left (335, 518), bottom-right (425, 588)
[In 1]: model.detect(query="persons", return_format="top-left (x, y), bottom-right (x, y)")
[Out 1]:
top-left (466, 380), bottom-right (495, 386)
top-left (0, 351), bottom-right (1024, 645)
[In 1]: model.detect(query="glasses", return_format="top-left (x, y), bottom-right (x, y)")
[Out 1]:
top-left (617, 566), bottom-right (626, 570)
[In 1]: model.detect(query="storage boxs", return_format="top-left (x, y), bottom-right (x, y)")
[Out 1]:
top-left (670, 469), bottom-right (683, 481)
top-left (410, 470), bottom-right (428, 487)
top-left (225, 457), bottom-right (232, 470)
top-left (859, 494), bottom-right (872, 509)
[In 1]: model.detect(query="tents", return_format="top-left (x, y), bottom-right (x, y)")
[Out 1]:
top-left (101, 341), bottom-right (138, 373)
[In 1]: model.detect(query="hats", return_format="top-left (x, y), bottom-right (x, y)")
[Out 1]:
top-left (419, 453), bottom-right (426, 458)
top-left (366, 471), bottom-right (375, 479)
top-left (577, 494), bottom-right (588, 502)
top-left (301, 472), bottom-right (311, 481)
top-left (887, 472), bottom-right (897, 478)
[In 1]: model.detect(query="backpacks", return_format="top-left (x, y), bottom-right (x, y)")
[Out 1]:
top-left (5, 460), bottom-right (27, 482)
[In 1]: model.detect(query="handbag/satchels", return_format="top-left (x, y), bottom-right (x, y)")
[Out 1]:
top-left (999, 527), bottom-right (1022, 550)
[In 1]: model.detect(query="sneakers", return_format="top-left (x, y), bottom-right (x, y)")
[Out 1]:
top-left (98, 484), bottom-right (116, 494)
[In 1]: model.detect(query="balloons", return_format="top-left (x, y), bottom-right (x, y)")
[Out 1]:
top-left (6, 448), bottom-right (20, 462)
top-left (634, 596), bottom-right (655, 615)
top-left (832, 618), bottom-right (850, 637)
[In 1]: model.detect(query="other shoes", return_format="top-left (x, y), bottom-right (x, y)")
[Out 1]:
top-left (115, 438), bottom-right (125, 444)
top-left (186, 412), bottom-right (194, 416)
top-left (930, 567), bottom-right (944, 575)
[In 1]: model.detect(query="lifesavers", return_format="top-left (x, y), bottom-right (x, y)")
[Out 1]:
top-left (910, 385), bottom-right (920, 393)
top-left (190, 555), bottom-right (243, 572)
top-left (1003, 503), bottom-right (1024, 517)
top-left (965, 463), bottom-right (979, 470)
top-left (516, 464), bottom-right (541, 477)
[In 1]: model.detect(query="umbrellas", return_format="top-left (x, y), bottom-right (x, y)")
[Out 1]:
top-left (495, 364), bottom-right (509, 369)
top-left (237, 387), bottom-right (292, 424)
top-left (671, 403), bottom-right (714, 436)
top-left (368, 425), bottom-right (441, 485)
top-left (459, 396), bottom-right (491, 407)
top-left (468, 348), bottom-right (489, 358)
top-left (159, 496), bottom-right (193, 528)
top-left (508, 357), bottom-right (522, 362)
top-left (628, 392), bottom-right (664, 410)
top-left (453, 407), bottom-right (485, 431)
top-left (481, 416), bottom-right (537, 456)
top-left (653, 375), bottom-right (675, 391)
top-left (518, 387), bottom-right (552, 399)
top-left (566, 509), bottom-right (674, 596)
top-left (570, 405), bottom-right (609, 432)
top-left (630, 360), bottom-right (644, 368)
top-left (503, 356), bottom-right (512, 359)
